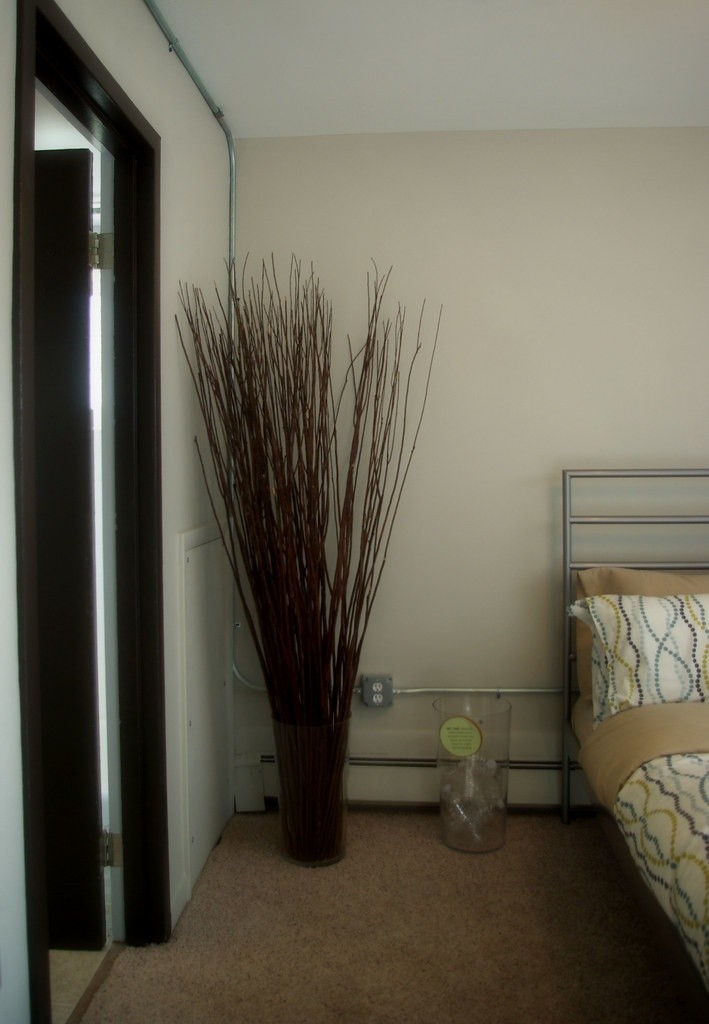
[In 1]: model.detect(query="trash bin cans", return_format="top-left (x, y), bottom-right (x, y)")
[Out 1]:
top-left (432, 693), bottom-right (513, 853)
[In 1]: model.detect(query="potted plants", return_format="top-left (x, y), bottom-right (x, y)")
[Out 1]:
top-left (175, 254), bottom-right (444, 868)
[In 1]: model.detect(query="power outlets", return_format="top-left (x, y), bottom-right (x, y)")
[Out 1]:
top-left (361, 673), bottom-right (394, 707)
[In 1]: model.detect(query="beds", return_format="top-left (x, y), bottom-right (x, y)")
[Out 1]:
top-left (564, 469), bottom-right (709, 991)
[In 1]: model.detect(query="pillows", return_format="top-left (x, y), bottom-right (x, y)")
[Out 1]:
top-left (569, 594), bottom-right (709, 728)
top-left (576, 568), bottom-right (709, 702)
